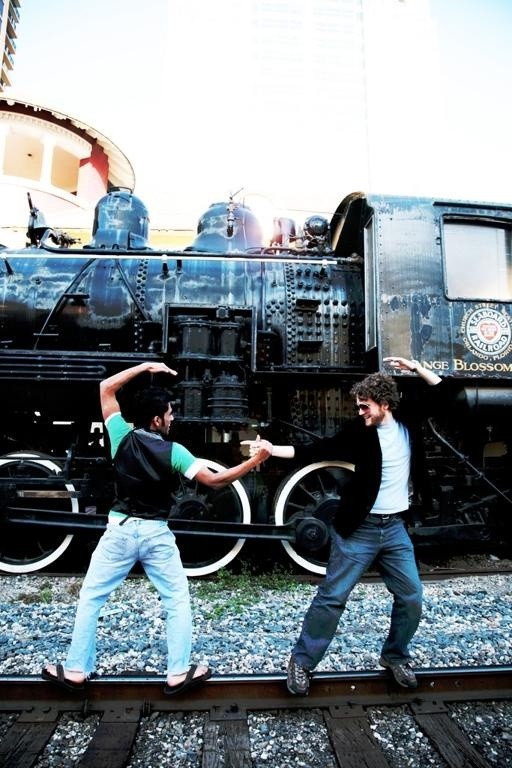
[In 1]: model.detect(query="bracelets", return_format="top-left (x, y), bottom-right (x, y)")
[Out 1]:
top-left (410, 364), bottom-right (419, 372)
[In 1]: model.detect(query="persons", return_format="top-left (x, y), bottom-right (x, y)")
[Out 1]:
top-left (43, 362), bottom-right (273, 687)
top-left (241, 357), bottom-right (457, 696)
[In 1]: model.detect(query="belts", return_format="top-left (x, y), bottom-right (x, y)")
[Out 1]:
top-left (367, 513), bottom-right (398, 520)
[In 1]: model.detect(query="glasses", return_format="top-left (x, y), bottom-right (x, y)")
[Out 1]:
top-left (354, 402), bottom-right (375, 410)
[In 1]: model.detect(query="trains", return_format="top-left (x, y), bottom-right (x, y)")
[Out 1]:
top-left (0, 186), bottom-right (512, 577)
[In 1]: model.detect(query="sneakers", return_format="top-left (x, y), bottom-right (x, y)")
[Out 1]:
top-left (378, 656), bottom-right (418, 688)
top-left (287, 654), bottom-right (311, 698)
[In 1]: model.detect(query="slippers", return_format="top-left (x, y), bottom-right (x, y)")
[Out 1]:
top-left (163, 665), bottom-right (212, 694)
top-left (43, 664), bottom-right (87, 690)
